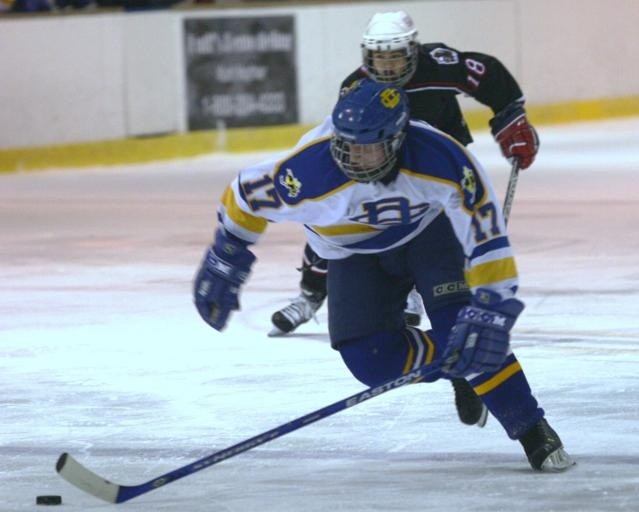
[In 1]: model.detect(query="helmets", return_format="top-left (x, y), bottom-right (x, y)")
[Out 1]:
top-left (357, 8), bottom-right (419, 92)
top-left (328, 79), bottom-right (412, 182)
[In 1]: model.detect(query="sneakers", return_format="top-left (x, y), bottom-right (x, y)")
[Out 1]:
top-left (404, 290), bottom-right (424, 325)
top-left (271, 283), bottom-right (327, 332)
top-left (519, 414), bottom-right (563, 469)
top-left (450, 383), bottom-right (484, 425)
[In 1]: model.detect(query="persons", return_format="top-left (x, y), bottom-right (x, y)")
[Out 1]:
top-left (270, 10), bottom-right (541, 333)
top-left (194, 77), bottom-right (564, 472)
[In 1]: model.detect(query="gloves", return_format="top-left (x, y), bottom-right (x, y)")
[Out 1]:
top-left (486, 101), bottom-right (542, 169)
top-left (193, 228), bottom-right (258, 331)
top-left (441, 287), bottom-right (526, 381)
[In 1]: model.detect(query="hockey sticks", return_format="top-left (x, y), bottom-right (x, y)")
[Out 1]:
top-left (55, 348), bottom-right (460, 503)
